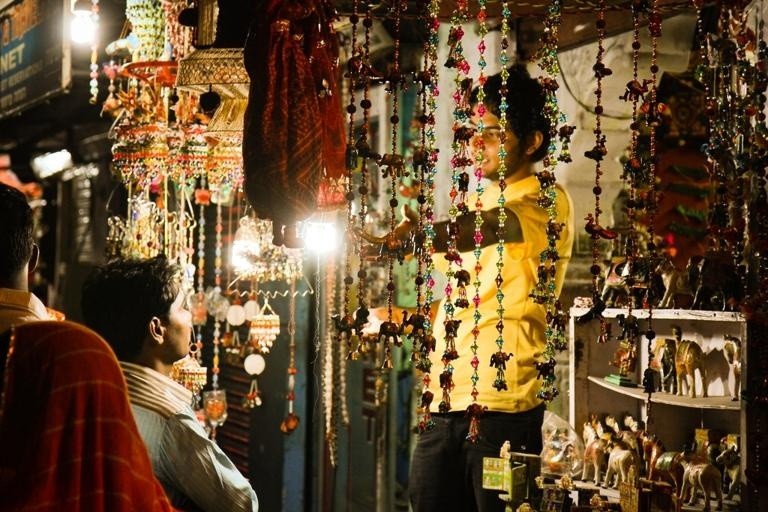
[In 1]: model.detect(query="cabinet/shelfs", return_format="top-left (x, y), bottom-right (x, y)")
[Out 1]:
top-left (569, 306), bottom-right (750, 511)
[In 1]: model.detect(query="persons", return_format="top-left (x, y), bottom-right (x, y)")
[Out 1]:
top-left (358, 73), bottom-right (575, 512)
top-left (0, 321), bottom-right (180, 512)
top-left (81, 254), bottom-right (260, 512)
top-left (0, 181), bottom-right (66, 333)
